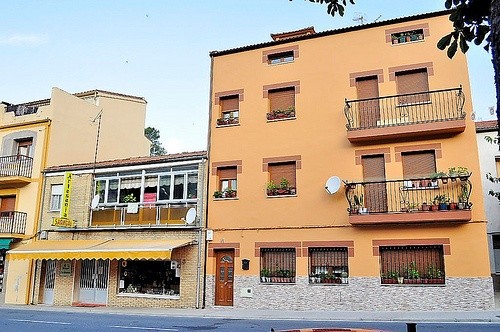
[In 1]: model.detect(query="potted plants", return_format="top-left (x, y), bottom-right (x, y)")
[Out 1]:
top-left (410, 30), bottom-right (417, 41)
top-left (458, 166), bottom-right (472, 181)
top-left (265, 178), bottom-right (296, 196)
top-left (260, 266), bottom-right (296, 283)
top-left (429, 172), bottom-right (438, 182)
top-left (438, 193), bottom-right (450, 210)
top-left (449, 191), bottom-right (458, 210)
top-left (352, 205), bottom-right (358, 214)
top-left (447, 167), bottom-right (459, 182)
top-left (437, 172), bottom-right (449, 184)
top-left (380, 260), bottom-right (445, 284)
top-left (405, 32), bottom-right (411, 42)
top-left (391, 34), bottom-right (399, 44)
top-left (399, 33), bottom-right (407, 43)
top-left (308, 270), bottom-right (348, 284)
top-left (400, 194), bottom-right (408, 212)
top-left (217, 115), bottom-right (239, 125)
top-left (458, 182), bottom-right (469, 209)
top-left (213, 185), bottom-right (233, 198)
top-left (428, 194), bottom-right (444, 211)
top-left (266, 104), bottom-right (295, 120)
top-left (416, 33), bottom-right (424, 40)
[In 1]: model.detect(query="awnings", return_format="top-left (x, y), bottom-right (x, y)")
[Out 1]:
top-left (5, 239), bottom-right (192, 261)
top-left (0, 239), bottom-right (16, 249)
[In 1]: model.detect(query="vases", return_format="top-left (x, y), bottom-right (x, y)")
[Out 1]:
top-left (232, 190), bottom-right (237, 198)
top-left (363, 207), bottom-right (368, 212)
top-left (421, 204), bottom-right (431, 211)
